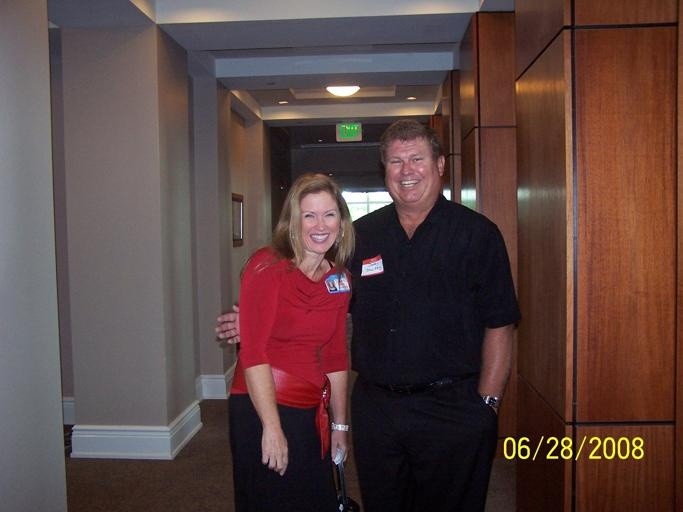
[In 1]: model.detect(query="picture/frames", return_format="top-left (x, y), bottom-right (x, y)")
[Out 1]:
top-left (231, 192), bottom-right (243, 248)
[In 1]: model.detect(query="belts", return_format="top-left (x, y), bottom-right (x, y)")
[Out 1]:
top-left (374, 378), bottom-right (452, 397)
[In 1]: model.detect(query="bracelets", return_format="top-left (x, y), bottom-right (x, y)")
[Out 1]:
top-left (330, 421), bottom-right (349, 431)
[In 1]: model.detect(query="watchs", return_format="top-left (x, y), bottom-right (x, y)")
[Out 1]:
top-left (482, 395), bottom-right (500, 408)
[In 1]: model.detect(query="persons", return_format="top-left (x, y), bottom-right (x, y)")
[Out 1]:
top-left (214, 117), bottom-right (518, 509)
top-left (228, 173), bottom-right (355, 511)
